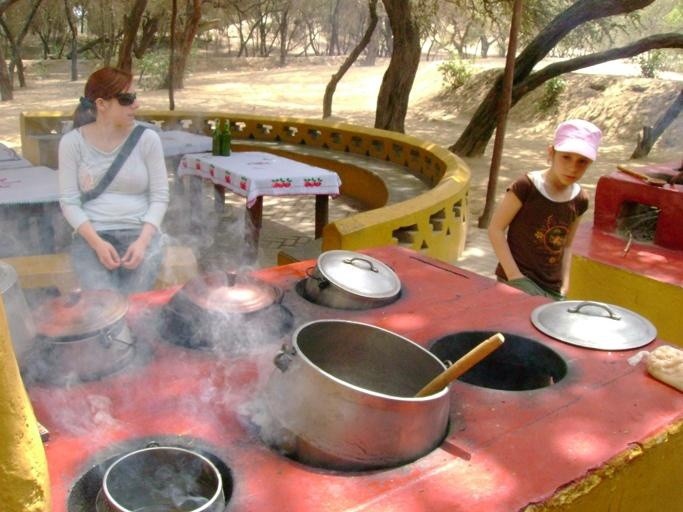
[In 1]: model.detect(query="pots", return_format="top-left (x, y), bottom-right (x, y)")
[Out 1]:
top-left (167, 271), bottom-right (292, 349)
top-left (259, 318), bottom-right (451, 472)
top-left (305, 248), bottom-right (400, 305)
top-left (25, 285), bottom-right (137, 385)
top-left (96, 441), bottom-right (226, 512)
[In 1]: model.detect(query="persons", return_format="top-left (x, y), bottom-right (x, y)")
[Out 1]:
top-left (58, 67), bottom-right (170, 290)
top-left (487, 118), bottom-right (602, 301)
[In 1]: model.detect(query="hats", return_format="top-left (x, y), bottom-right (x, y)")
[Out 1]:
top-left (551, 118), bottom-right (603, 162)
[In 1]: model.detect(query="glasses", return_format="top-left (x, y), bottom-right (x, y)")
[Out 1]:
top-left (108, 91), bottom-right (136, 106)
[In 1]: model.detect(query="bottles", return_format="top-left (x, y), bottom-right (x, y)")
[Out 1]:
top-left (211, 119), bottom-right (232, 156)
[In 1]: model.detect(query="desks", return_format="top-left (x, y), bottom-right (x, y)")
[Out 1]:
top-left (0, 141), bottom-right (63, 254)
top-left (159, 131), bottom-right (214, 197)
top-left (180, 150), bottom-right (340, 266)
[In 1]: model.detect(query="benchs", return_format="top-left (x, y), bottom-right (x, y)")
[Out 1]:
top-left (207, 139), bottom-right (430, 273)
top-left (1, 245), bottom-right (197, 294)
